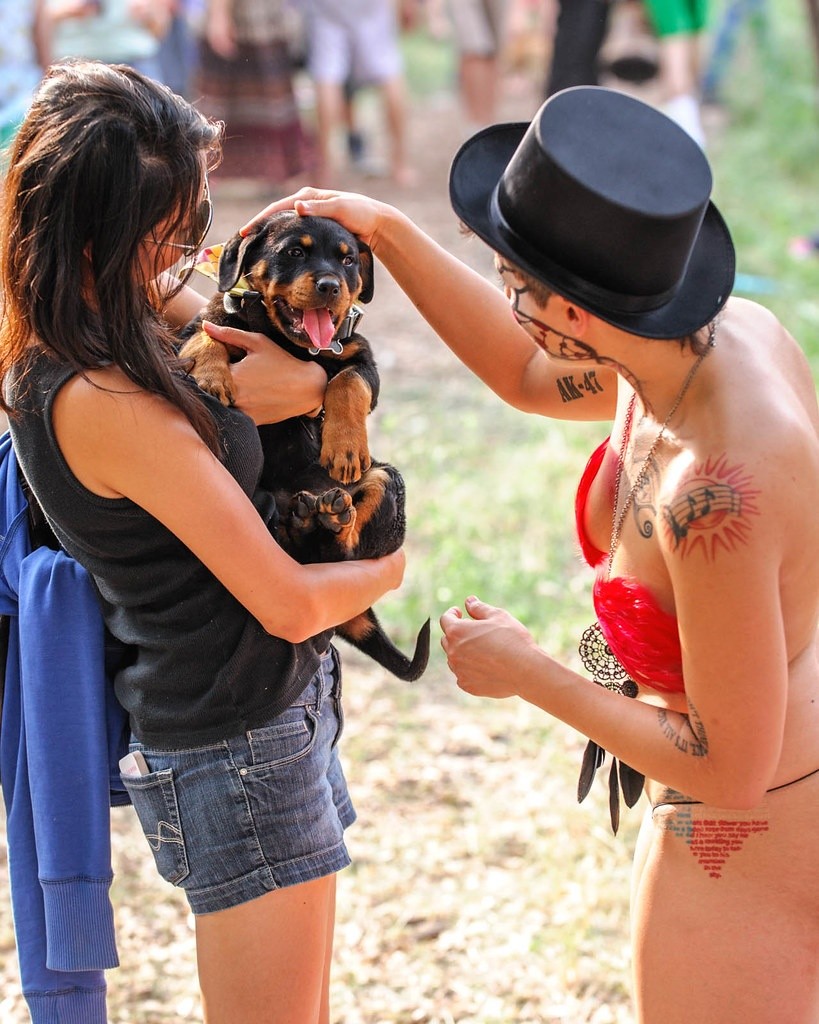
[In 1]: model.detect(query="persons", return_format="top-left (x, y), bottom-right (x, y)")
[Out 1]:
top-left (0, 56), bottom-right (408, 1024)
top-left (0, 0), bottom-right (702, 185)
top-left (239, 83), bottom-right (819, 1024)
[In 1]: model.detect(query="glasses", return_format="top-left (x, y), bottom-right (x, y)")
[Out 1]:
top-left (139, 170), bottom-right (214, 257)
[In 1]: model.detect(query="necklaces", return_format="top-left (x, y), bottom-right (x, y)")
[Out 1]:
top-left (578, 319), bottom-right (716, 836)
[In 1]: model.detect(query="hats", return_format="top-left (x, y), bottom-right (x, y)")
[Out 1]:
top-left (450, 85), bottom-right (736, 339)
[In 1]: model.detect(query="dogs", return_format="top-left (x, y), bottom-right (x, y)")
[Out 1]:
top-left (176, 209), bottom-right (432, 683)
